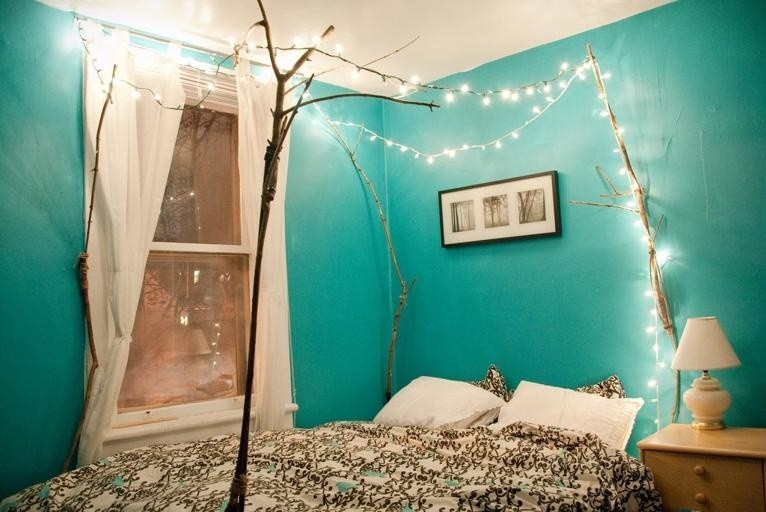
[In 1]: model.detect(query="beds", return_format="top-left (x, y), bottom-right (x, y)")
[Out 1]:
top-left (1, 420), bottom-right (663, 512)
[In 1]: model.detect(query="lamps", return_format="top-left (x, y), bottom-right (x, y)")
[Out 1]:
top-left (670, 315), bottom-right (743, 432)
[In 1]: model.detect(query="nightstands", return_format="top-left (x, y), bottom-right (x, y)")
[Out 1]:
top-left (637, 421), bottom-right (766, 512)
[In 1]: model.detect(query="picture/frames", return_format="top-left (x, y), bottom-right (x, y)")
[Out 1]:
top-left (438, 170), bottom-right (562, 248)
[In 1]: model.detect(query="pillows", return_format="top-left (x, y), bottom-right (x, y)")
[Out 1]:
top-left (369, 362), bottom-right (645, 454)
